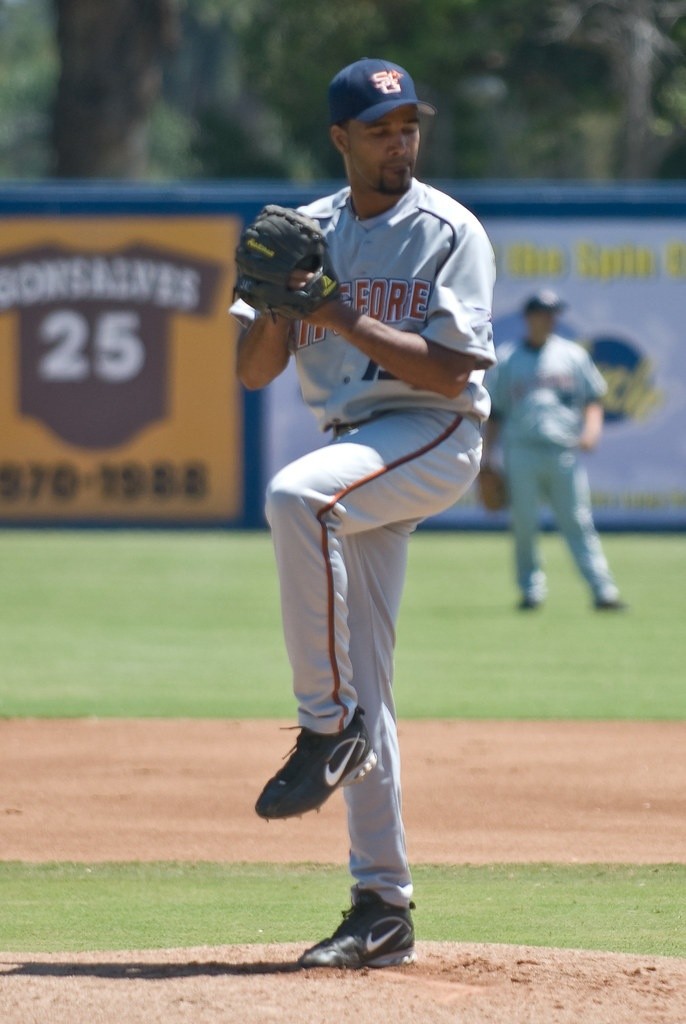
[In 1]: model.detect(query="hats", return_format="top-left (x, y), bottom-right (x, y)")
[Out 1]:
top-left (327, 57), bottom-right (439, 124)
top-left (522, 289), bottom-right (568, 312)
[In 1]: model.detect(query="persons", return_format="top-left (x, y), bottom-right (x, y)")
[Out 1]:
top-left (225, 54), bottom-right (497, 969)
top-left (478, 289), bottom-right (625, 613)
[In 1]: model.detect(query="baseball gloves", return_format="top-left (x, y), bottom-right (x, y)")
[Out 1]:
top-left (476, 464), bottom-right (508, 511)
top-left (231, 202), bottom-right (341, 324)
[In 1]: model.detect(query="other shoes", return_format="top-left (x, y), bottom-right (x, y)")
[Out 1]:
top-left (592, 589), bottom-right (628, 610)
top-left (516, 587), bottom-right (545, 610)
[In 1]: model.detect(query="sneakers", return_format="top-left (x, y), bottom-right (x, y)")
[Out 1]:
top-left (298, 890), bottom-right (419, 968)
top-left (254, 703), bottom-right (378, 819)
